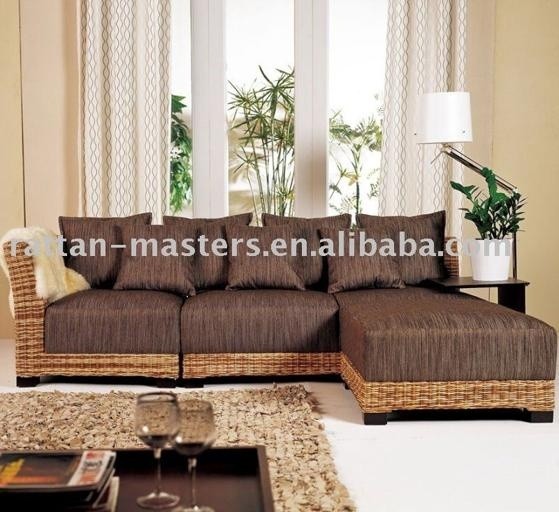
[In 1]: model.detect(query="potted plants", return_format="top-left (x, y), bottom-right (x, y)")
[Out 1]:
top-left (450, 164), bottom-right (528, 284)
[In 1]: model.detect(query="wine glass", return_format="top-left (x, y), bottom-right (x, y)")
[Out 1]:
top-left (134, 392), bottom-right (217, 511)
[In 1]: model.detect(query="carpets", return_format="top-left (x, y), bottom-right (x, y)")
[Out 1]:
top-left (1, 384), bottom-right (355, 511)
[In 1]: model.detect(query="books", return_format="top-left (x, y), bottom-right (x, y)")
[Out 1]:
top-left (0, 450), bottom-right (120, 512)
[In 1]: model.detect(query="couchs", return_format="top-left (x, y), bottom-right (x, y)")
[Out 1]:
top-left (1, 211), bottom-right (558, 426)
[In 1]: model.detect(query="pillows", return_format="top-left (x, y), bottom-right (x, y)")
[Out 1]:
top-left (112, 223), bottom-right (408, 297)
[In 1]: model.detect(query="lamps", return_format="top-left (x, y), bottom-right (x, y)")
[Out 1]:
top-left (415, 91), bottom-right (520, 282)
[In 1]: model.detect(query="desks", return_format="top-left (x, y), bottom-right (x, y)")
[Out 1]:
top-left (427, 276), bottom-right (530, 313)
